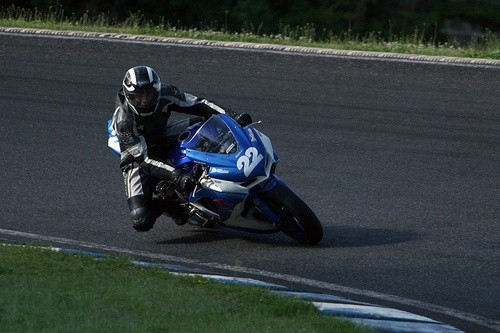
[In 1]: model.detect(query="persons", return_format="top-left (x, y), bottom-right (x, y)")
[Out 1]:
top-left (111, 66), bottom-right (252, 232)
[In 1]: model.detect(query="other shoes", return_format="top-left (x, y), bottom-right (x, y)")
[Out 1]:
top-left (157, 193), bottom-right (187, 225)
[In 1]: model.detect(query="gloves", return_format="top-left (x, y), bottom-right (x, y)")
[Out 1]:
top-left (170, 169), bottom-right (198, 192)
top-left (225, 108), bottom-right (253, 127)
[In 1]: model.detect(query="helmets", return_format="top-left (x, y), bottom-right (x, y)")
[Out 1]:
top-left (123, 66), bottom-right (161, 116)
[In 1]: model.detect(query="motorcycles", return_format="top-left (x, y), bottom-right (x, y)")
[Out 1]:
top-left (107, 113), bottom-right (324, 245)
